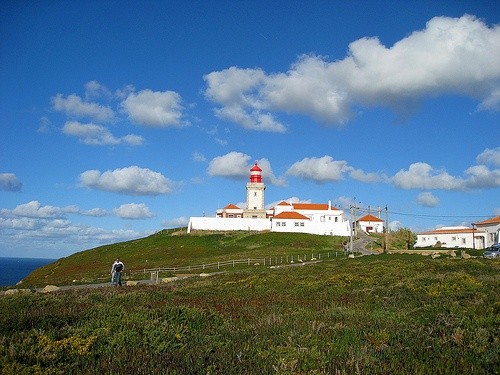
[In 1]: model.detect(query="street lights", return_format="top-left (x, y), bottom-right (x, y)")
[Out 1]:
top-left (472, 223), bottom-right (475, 249)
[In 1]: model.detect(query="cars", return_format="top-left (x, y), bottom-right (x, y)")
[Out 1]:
top-left (483, 246), bottom-right (500, 260)
top-left (492, 243), bottom-right (500, 247)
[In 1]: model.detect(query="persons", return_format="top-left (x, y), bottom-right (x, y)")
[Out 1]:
top-left (110, 260), bottom-right (119, 283)
top-left (110, 257), bottom-right (126, 287)
